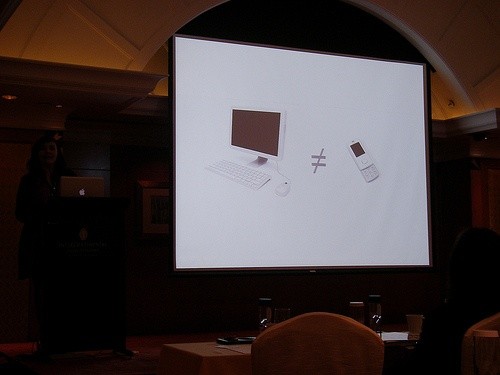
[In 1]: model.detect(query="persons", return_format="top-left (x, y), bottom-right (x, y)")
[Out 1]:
top-left (385, 227), bottom-right (500, 375)
top-left (17, 135), bottom-right (69, 261)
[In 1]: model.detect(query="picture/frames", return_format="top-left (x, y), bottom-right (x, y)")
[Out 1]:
top-left (135, 181), bottom-right (170, 242)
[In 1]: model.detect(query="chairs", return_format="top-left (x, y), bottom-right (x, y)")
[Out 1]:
top-left (460, 312), bottom-right (500, 375)
top-left (250, 311), bottom-right (384, 375)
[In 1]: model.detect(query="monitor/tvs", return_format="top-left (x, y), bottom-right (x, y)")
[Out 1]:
top-left (227, 108), bottom-right (281, 162)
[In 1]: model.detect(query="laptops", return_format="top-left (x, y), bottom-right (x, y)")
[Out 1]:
top-left (58, 175), bottom-right (105, 198)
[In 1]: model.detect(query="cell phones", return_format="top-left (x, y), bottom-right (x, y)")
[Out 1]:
top-left (347, 140), bottom-right (380, 184)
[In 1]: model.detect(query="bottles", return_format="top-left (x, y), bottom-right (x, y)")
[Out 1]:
top-left (257, 298), bottom-right (272, 330)
top-left (367, 294), bottom-right (383, 333)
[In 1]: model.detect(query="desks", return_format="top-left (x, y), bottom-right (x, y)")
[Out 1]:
top-left (159, 330), bottom-right (419, 375)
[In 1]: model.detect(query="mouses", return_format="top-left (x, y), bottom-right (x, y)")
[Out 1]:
top-left (276, 175), bottom-right (290, 197)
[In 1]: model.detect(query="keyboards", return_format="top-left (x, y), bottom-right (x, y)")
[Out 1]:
top-left (205, 158), bottom-right (270, 191)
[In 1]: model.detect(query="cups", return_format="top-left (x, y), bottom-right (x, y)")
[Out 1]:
top-left (406, 314), bottom-right (424, 336)
top-left (349, 301), bottom-right (365, 326)
top-left (273, 308), bottom-right (291, 323)
